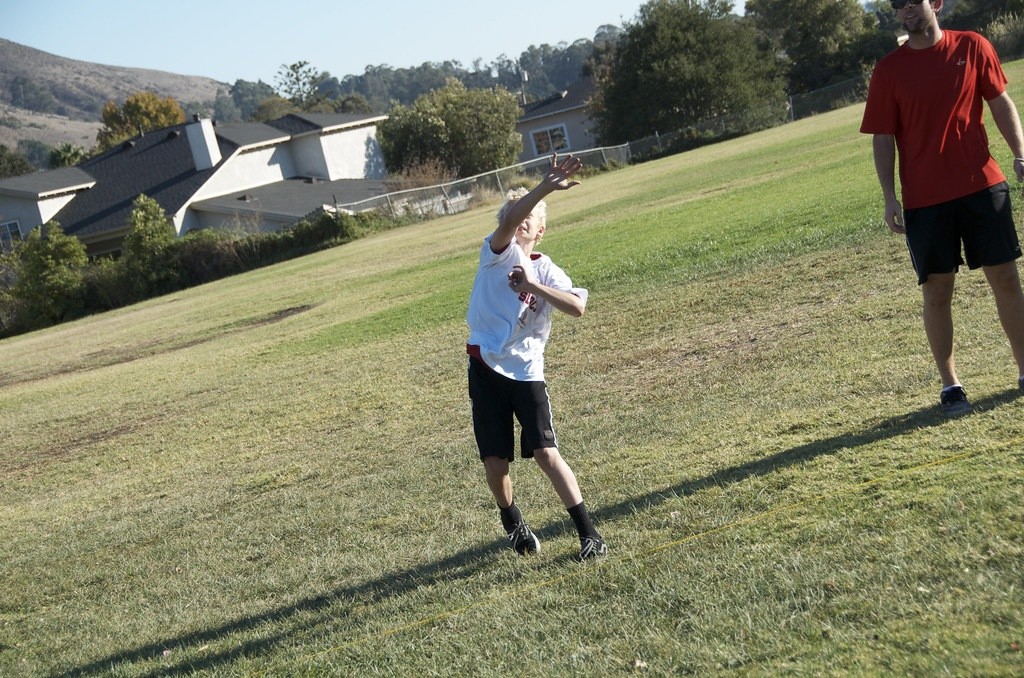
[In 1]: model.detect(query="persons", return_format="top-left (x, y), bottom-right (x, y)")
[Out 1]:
top-left (860, 1), bottom-right (1024, 418)
top-left (466, 152), bottom-right (606, 563)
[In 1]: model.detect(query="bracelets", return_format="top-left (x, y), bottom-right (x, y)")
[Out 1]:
top-left (1014, 158), bottom-right (1024, 162)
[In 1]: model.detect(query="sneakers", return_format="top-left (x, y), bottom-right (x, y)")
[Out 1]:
top-left (501, 505), bottom-right (541, 558)
top-left (1018, 378), bottom-right (1024, 396)
top-left (579, 536), bottom-right (607, 562)
top-left (940, 386), bottom-right (972, 419)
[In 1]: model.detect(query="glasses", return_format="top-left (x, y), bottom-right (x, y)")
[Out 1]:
top-left (892, 0), bottom-right (924, 9)
